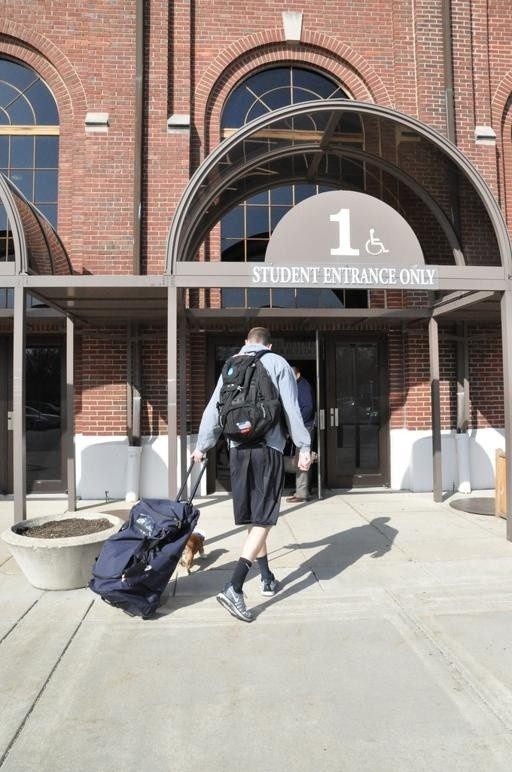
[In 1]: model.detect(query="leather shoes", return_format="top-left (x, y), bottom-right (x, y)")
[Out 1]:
top-left (285, 497), bottom-right (309, 502)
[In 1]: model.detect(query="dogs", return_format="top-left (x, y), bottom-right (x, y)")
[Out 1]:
top-left (178, 533), bottom-right (208, 576)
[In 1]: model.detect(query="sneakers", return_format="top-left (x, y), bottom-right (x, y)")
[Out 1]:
top-left (261, 579), bottom-right (279, 596)
top-left (217, 582), bottom-right (254, 622)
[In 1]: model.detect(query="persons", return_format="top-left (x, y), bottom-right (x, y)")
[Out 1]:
top-left (190, 326), bottom-right (312, 623)
top-left (286, 366), bottom-right (316, 503)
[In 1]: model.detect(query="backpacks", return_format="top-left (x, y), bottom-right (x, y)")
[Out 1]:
top-left (217, 350), bottom-right (280, 442)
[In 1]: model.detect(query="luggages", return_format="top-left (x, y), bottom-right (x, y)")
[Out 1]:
top-left (88, 456), bottom-right (208, 620)
